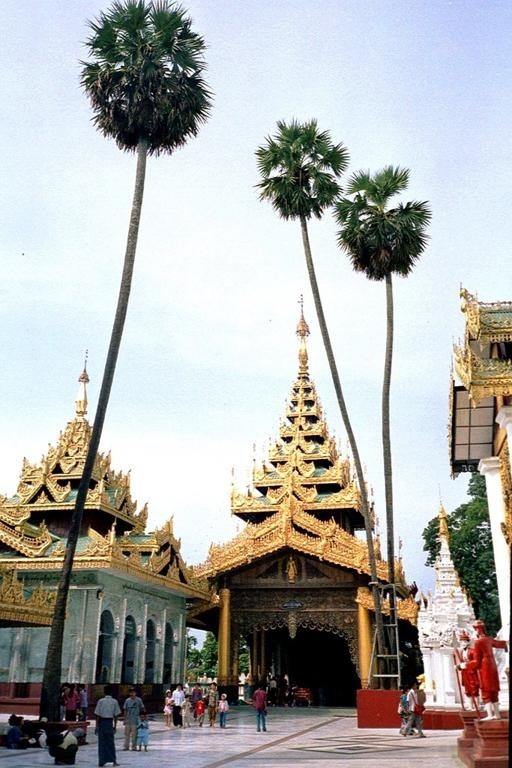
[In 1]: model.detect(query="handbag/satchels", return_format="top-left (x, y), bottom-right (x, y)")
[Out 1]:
top-left (46, 734), bottom-right (63, 746)
top-left (414, 704), bottom-right (425, 716)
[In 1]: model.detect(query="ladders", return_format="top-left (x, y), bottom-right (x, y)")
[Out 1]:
top-left (367, 582), bottom-right (402, 690)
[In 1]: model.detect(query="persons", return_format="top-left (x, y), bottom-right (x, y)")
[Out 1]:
top-left (402, 683), bottom-right (427, 737)
top-left (285, 556), bottom-right (298, 584)
top-left (397, 685), bottom-right (415, 734)
top-left (243, 671), bottom-right (291, 708)
top-left (452, 619), bottom-right (509, 722)
top-left (453, 629), bottom-right (481, 698)
top-left (180, 695), bottom-right (195, 729)
top-left (218, 692), bottom-right (231, 728)
top-left (195, 694), bottom-right (206, 727)
top-left (204, 682), bottom-right (220, 727)
top-left (164, 688), bottom-right (174, 728)
top-left (59, 681), bottom-right (90, 722)
top-left (252, 681), bottom-right (268, 732)
top-left (191, 682), bottom-right (203, 722)
top-left (49, 727), bottom-right (87, 765)
top-left (7, 712), bottom-right (29, 750)
top-left (94, 685), bottom-right (123, 767)
top-left (122, 686), bottom-right (148, 751)
top-left (168, 683), bottom-right (186, 728)
top-left (136, 711), bottom-right (151, 751)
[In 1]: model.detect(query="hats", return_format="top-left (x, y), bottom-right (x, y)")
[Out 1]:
top-left (72, 728), bottom-right (84, 737)
